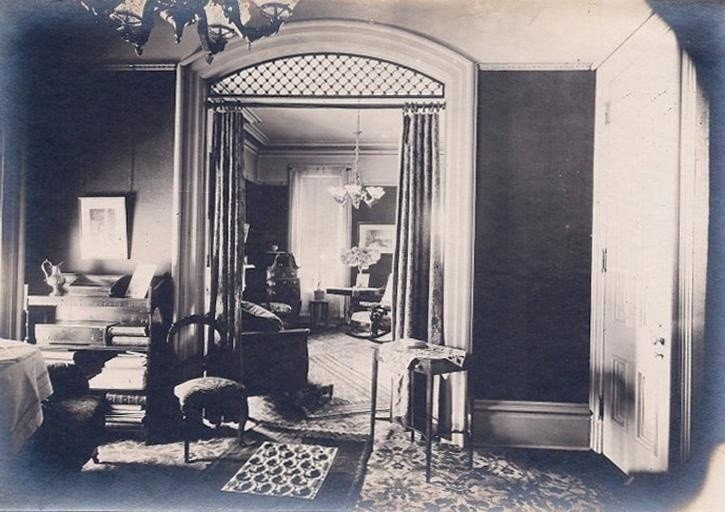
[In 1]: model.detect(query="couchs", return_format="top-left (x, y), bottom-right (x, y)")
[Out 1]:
top-left (240, 299), bottom-right (310, 400)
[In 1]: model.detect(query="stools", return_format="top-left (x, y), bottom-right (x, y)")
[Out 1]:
top-left (309, 300), bottom-right (328, 332)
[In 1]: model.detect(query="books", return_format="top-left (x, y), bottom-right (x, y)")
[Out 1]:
top-left (109, 320), bottom-right (151, 345)
top-left (88, 351), bottom-right (147, 390)
top-left (42, 350), bottom-right (80, 364)
top-left (105, 393), bottom-right (147, 427)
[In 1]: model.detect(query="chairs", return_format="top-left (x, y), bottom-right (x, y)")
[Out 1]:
top-left (167, 316), bottom-right (249, 464)
top-left (35, 363), bottom-right (106, 465)
top-left (345, 272), bottom-right (393, 344)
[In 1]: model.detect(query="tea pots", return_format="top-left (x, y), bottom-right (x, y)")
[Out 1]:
top-left (41, 261), bottom-right (67, 297)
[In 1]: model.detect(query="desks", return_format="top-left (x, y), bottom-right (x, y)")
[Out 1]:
top-left (326, 287), bottom-right (380, 325)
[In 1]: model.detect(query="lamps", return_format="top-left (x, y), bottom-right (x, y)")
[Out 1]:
top-left (330, 110), bottom-right (386, 210)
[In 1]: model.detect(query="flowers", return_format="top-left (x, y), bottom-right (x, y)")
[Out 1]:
top-left (338, 242), bottom-right (382, 273)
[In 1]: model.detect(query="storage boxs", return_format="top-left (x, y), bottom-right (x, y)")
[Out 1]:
top-left (35, 319), bottom-right (120, 348)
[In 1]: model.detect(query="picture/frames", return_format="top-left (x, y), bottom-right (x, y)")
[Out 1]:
top-left (357, 221), bottom-right (396, 256)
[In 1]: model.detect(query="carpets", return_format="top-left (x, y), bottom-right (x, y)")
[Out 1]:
top-left (285, 329), bottom-right (398, 418)
top-left (161, 420), bottom-right (373, 512)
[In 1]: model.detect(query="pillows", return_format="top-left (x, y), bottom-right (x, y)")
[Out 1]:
top-left (261, 302), bottom-right (291, 316)
top-left (240, 298), bottom-right (282, 332)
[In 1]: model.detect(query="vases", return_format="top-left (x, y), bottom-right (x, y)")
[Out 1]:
top-left (356, 273), bottom-right (370, 288)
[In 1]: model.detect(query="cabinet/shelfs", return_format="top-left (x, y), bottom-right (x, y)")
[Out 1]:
top-left (21, 273), bottom-right (170, 440)
top-left (368, 338), bottom-right (474, 482)
